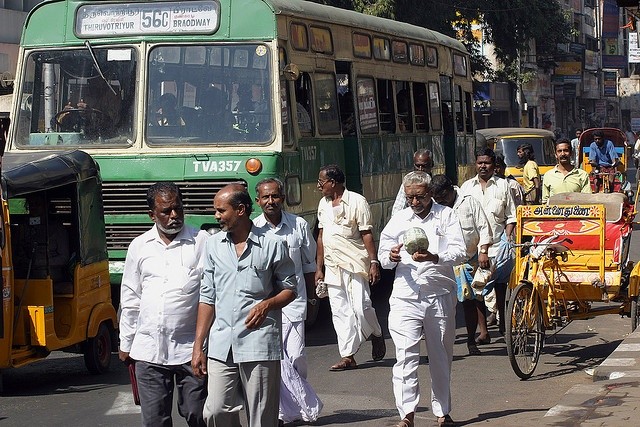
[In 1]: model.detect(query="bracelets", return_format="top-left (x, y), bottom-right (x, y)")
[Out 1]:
top-left (370, 259), bottom-right (380, 266)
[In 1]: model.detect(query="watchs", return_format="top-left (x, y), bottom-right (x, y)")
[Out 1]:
top-left (479, 248), bottom-right (488, 254)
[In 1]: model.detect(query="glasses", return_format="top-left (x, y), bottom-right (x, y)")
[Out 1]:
top-left (316, 179), bottom-right (332, 188)
top-left (404, 191), bottom-right (429, 201)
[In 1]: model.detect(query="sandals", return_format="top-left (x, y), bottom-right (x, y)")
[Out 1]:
top-left (371, 332), bottom-right (386, 361)
top-left (397, 412), bottom-right (414, 427)
top-left (329, 357), bottom-right (359, 372)
top-left (437, 415), bottom-right (455, 427)
top-left (466, 340), bottom-right (481, 356)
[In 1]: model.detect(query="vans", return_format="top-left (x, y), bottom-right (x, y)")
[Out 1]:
top-left (475, 124), bottom-right (559, 191)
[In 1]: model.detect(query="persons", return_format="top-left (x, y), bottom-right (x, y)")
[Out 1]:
top-left (391, 147), bottom-right (434, 220)
top-left (587, 129), bottom-right (621, 194)
top-left (540, 137), bottom-right (592, 205)
top-left (493, 152), bottom-right (526, 206)
top-left (375, 169), bottom-right (468, 425)
top-left (631, 131), bottom-right (640, 168)
top-left (251, 175), bottom-right (325, 425)
top-left (153, 74), bottom-right (461, 142)
top-left (57, 60), bottom-right (122, 131)
top-left (314, 164), bottom-right (386, 372)
top-left (460, 147), bottom-right (517, 344)
top-left (516, 142), bottom-right (540, 205)
top-left (429, 173), bottom-right (494, 357)
top-left (13, 193), bottom-right (71, 281)
top-left (191, 181), bottom-right (299, 426)
top-left (624, 125), bottom-right (635, 154)
top-left (570, 128), bottom-right (582, 167)
top-left (115, 178), bottom-right (211, 427)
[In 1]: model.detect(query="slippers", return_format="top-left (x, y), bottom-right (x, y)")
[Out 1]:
top-left (475, 336), bottom-right (490, 345)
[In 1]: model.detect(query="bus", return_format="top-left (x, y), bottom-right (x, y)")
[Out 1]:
top-left (2, 0), bottom-right (475, 332)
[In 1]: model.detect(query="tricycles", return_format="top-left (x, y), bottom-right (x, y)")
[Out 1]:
top-left (577, 126), bottom-right (640, 219)
top-left (505, 193), bottom-right (640, 382)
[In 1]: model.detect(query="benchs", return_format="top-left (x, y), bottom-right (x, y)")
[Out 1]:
top-left (16, 238), bottom-right (77, 294)
top-left (531, 193), bottom-right (631, 249)
